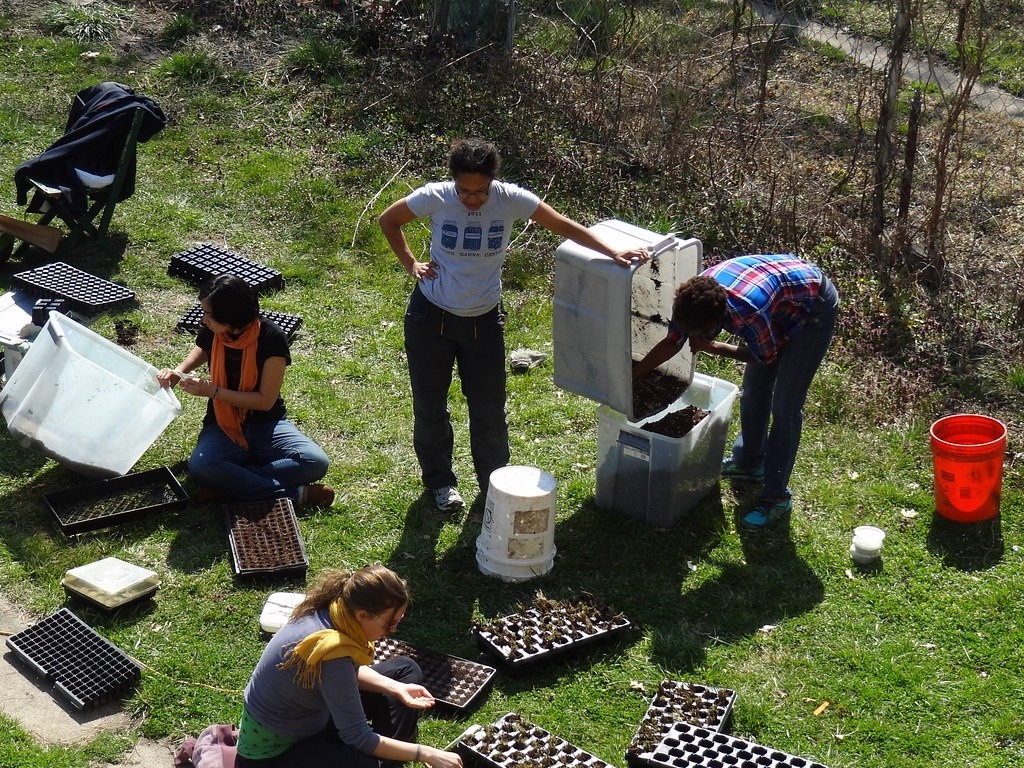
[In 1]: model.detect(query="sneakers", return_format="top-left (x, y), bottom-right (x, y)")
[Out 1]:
top-left (433, 485), bottom-right (463, 511)
top-left (718, 446), bottom-right (765, 483)
top-left (740, 495), bottom-right (792, 529)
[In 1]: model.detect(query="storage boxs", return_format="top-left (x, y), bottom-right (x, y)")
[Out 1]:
top-left (0, 309), bottom-right (184, 483)
top-left (1, 288), bottom-right (43, 383)
top-left (550, 221), bottom-right (706, 422)
top-left (591, 374), bottom-right (749, 530)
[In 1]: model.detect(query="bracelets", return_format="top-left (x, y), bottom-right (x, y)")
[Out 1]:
top-left (213, 387), bottom-right (219, 399)
top-left (414, 744), bottom-right (421, 763)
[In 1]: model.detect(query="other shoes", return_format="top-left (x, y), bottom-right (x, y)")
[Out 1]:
top-left (298, 484), bottom-right (335, 507)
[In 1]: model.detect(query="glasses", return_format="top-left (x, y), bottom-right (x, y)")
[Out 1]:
top-left (357, 608), bottom-right (405, 632)
top-left (453, 178), bottom-right (491, 198)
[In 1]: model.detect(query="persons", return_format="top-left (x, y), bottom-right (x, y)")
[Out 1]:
top-left (234, 567), bottom-right (465, 768)
top-left (632, 255), bottom-right (840, 531)
top-left (156, 274), bottom-right (334, 509)
top-left (378, 140), bottom-right (648, 511)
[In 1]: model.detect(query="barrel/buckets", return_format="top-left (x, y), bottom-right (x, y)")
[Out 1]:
top-left (930, 414), bottom-right (1007, 523)
top-left (473, 465), bottom-right (557, 583)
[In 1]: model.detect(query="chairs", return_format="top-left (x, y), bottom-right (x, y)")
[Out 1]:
top-left (13, 84), bottom-right (172, 260)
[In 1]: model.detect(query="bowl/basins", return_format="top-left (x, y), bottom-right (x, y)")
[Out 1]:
top-left (850, 526), bottom-right (885, 563)
